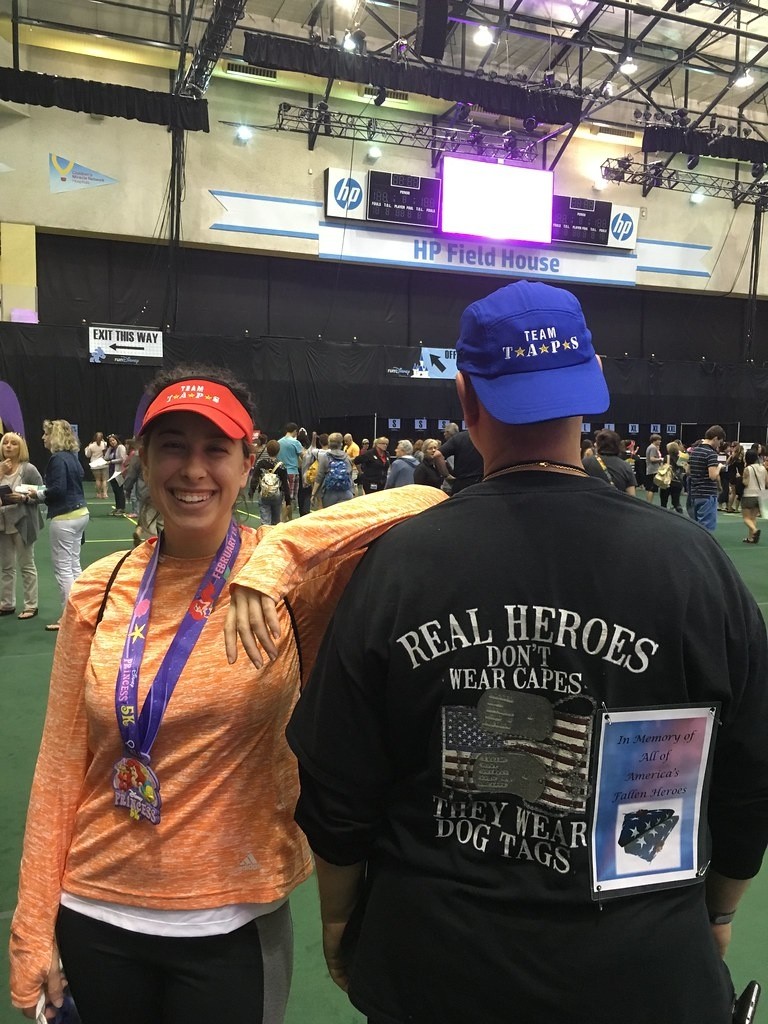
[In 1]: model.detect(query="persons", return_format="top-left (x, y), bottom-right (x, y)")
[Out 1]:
top-left (85, 423), bottom-right (459, 547)
top-left (433, 430), bottom-right (483, 496)
top-left (581, 428), bottom-right (768, 543)
top-left (688, 425), bottom-right (726, 532)
top-left (9, 367), bottom-right (451, 1024)
top-left (0, 431), bottom-right (44, 619)
top-left (581, 429), bottom-right (638, 496)
top-left (28, 419), bottom-right (90, 631)
top-left (284, 279), bottom-right (768, 1024)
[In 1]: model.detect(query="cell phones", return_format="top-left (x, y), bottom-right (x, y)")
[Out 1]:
top-left (733, 980), bottom-right (761, 1024)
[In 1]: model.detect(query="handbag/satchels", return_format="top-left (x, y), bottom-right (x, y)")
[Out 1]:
top-left (306, 460), bottom-right (318, 484)
top-left (653, 455), bottom-right (672, 489)
top-left (758, 489), bottom-right (768, 519)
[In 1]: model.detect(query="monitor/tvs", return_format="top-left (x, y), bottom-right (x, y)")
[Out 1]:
top-left (440, 156), bottom-right (554, 245)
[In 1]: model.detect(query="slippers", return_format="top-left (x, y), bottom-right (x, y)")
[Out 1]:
top-left (0, 608), bottom-right (15, 616)
top-left (18, 608), bottom-right (38, 619)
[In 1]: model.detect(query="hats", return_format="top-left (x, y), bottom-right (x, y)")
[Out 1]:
top-left (362, 439), bottom-right (370, 443)
top-left (455, 280), bottom-right (610, 424)
top-left (136, 376), bottom-right (255, 440)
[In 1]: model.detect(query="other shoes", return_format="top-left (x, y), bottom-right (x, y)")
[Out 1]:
top-left (114, 509), bottom-right (126, 515)
top-left (752, 530), bottom-right (760, 543)
top-left (727, 510), bottom-right (734, 513)
top-left (133, 532), bottom-right (141, 547)
top-left (725, 508), bottom-right (735, 511)
top-left (717, 509), bottom-right (726, 511)
top-left (743, 538), bottom-right (754, 543)
top-left (733, 511), bottom-right (740, 513)
top-left (126, 513), bottom-right (138, 517)
top-left (108, 509), bottom-right (117, 515)
top-left (46, 622), bottom-right (60, 631)
top-left (97, 494), bottom-right (103, 500)
top-left (103, 494), bottom-right (108, 498)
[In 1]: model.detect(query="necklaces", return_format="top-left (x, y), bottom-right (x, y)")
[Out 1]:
top-left (483, 459), bottom-right (590, 481)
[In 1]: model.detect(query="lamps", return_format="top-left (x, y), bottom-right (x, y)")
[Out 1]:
top-left (238, 125), bottom-right (251, 140)
top-left (743, 128), bottom-right (751, 136)
top-left (563, 82), bottom-right (570, 90)
top-left (523, 116), bottom-right (538, 133)
top-left (717, 123), bottom-right (726, 132)
top-left (687, 156), bottom-right (699, 168)
top-left (751, 163), bottom-right (767, 178)
top-left (476, 67), bottom-right (485, 76)
top-left (544, 70), bottom-right (554, 86)
top-left (327, 34), bottom-right (337, 44)
top-left (594, 88), bottom-right (600, 98)
top-left (634, 105), bottom-right (691, 124)
top-left (373, 87), bottom-right (386, 107)
top-left (458, 107), bottom-right (470, 119)
top-left (489, 70), bottom-right (497, 78)
top-left (344, 28), bottom-right (365, 49)
top-left (729, 126), bottom-right (736, 134)
top-left (584, 86), bottom-right (591, 95)
top-left (573, 85), bottom-right (581, 93)
top-left (368, 147), bottom-right (382, 160)
top-left (313, 32), bottom-right (322, 42)
top-left (604, 90), bottom-right (609, 100)
top-left (554, 80), bottom-right (561, 88)
top-left (649, 161), bottom-right (665, 170)
top-left (506, 72), bottom-right (513, 81)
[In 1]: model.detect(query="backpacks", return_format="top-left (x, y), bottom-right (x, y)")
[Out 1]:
top-left (259, 461), bottom-right (282, 500)
top-left (325, 452), bottom-right (350, 491)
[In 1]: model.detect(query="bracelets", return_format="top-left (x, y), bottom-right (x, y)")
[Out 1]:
top-left (710, 910), bottom-right (735, 924)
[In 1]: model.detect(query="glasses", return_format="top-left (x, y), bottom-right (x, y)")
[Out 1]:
top-left (11, 432), bottom-right (23, 440)
top-left (108, 434), bottom-right (116, 437)
